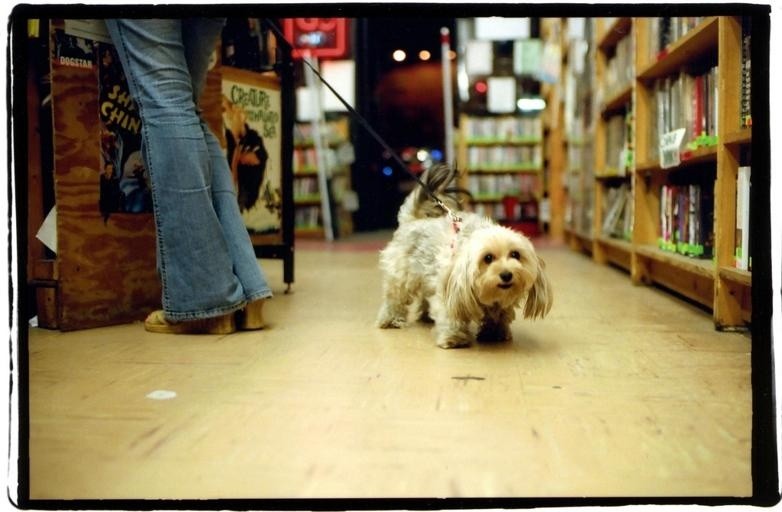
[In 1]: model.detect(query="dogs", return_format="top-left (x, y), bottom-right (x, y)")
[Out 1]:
top-left (376, 159), bottom-right (553, 349)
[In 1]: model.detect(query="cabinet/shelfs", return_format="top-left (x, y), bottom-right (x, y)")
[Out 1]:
top-left (458, 6), bottom-right (752, 333)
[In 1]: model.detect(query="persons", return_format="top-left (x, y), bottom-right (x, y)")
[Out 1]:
top-left (100, 20), bottom-right (274, 336)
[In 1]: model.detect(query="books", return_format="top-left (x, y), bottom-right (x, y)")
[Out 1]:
top-left (649, 17), bottom-right (717, 260)
top-left (732, 38), bottom-right (751, 271)
top-left (562, 43), bottom-right (594, 236)
top-left (463, 116), bottom-right (543, 223)
top-left (290, 130), bottom-right (323, 231)
top-left (599, 33), bottom-right (634, 241)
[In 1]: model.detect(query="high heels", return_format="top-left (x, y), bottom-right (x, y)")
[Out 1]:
top-left (144, 299), bottom-right (266, 335)
